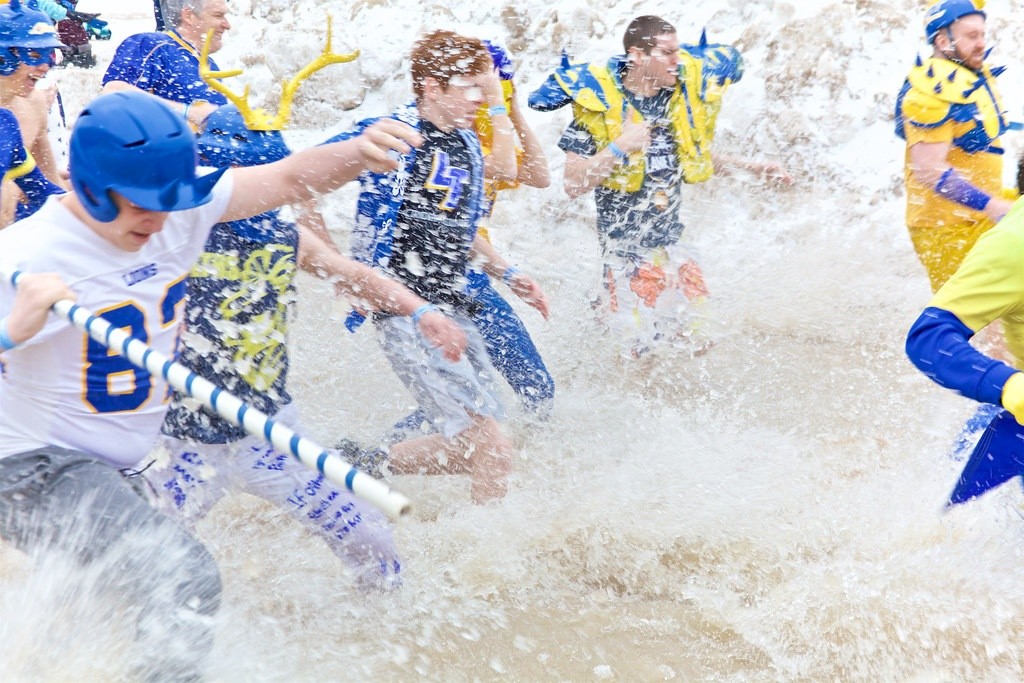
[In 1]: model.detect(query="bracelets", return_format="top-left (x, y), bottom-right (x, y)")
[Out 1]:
top-left (0, 327), bottom-right (17, 350)
top-left (409, 305), bottom-right (439, 320)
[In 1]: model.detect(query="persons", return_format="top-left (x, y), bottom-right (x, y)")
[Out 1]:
top-left (0, 89), bottom-right (428, 681)
top-left (156, 104), bottom-right (472, 605)
top-left (555, 16), bottom-right (719, 400)
top-left (283, 29), bottom-right (545, 482)
top-left (0, 3), bottom-right (60, 229)
top-left (350, 37), bottom-right (569, 457)
top-left (103, 2), bottom-right (234, 124)
top-left (891, 0), bottom-right (1018, 293)
top-left (901, 134), bottom-right (1024, 434)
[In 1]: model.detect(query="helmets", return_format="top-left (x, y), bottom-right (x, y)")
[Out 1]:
top-left (192, 102), bottom-right (291, 167)
top-left (925, 0), bottom-right (986, 46)
top-left (69, 89), bottom-right (236, 224)
top-left (0, 0), bottom-right (68, 76)
